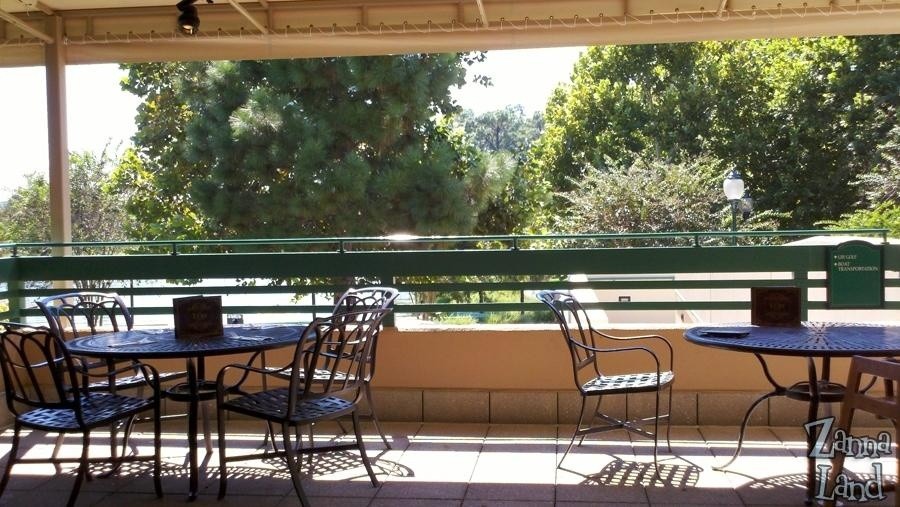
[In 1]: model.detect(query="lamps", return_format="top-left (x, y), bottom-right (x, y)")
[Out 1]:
top-left (175, 0), bottom-right (201, 36)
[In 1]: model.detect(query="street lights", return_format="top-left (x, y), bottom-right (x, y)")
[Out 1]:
top-left (723, 168), bottom-right (744, 246)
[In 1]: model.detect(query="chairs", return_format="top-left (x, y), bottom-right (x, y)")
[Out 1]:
top-left (0, 288), bottom-right (401, 507)
top-left (537, 289), bottom-right (703, 490)
top-left (824, 356), bottom-right (900, 507)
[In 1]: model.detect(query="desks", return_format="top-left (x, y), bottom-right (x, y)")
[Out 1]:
top-left (686, 326), bottom-right (900, 507)
